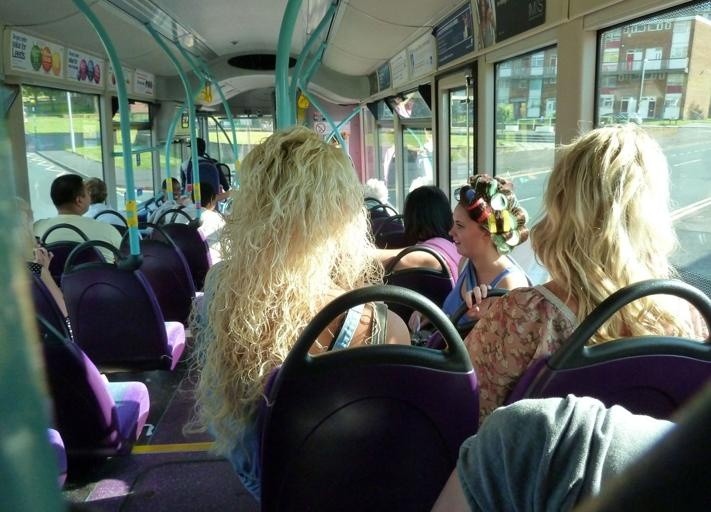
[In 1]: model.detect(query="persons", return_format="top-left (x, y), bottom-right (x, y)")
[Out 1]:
top-left (375, 172), bottom-right (533, 333)
top-left (33, 136), bottom-right (232, 265)
top-left (22, 194), bottom-right (72, 341)
top-left (427, 377), bottom-right (711, 512)
top-left (162, 122), bottom-right (410, 499)
top-left (464, 121), bottom-right (711, 429)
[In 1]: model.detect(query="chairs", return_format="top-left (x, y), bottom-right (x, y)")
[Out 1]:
top-left (31, 154), bottom-right (233, 487)
top-left (249, 197), bottom-right (711, 510)
